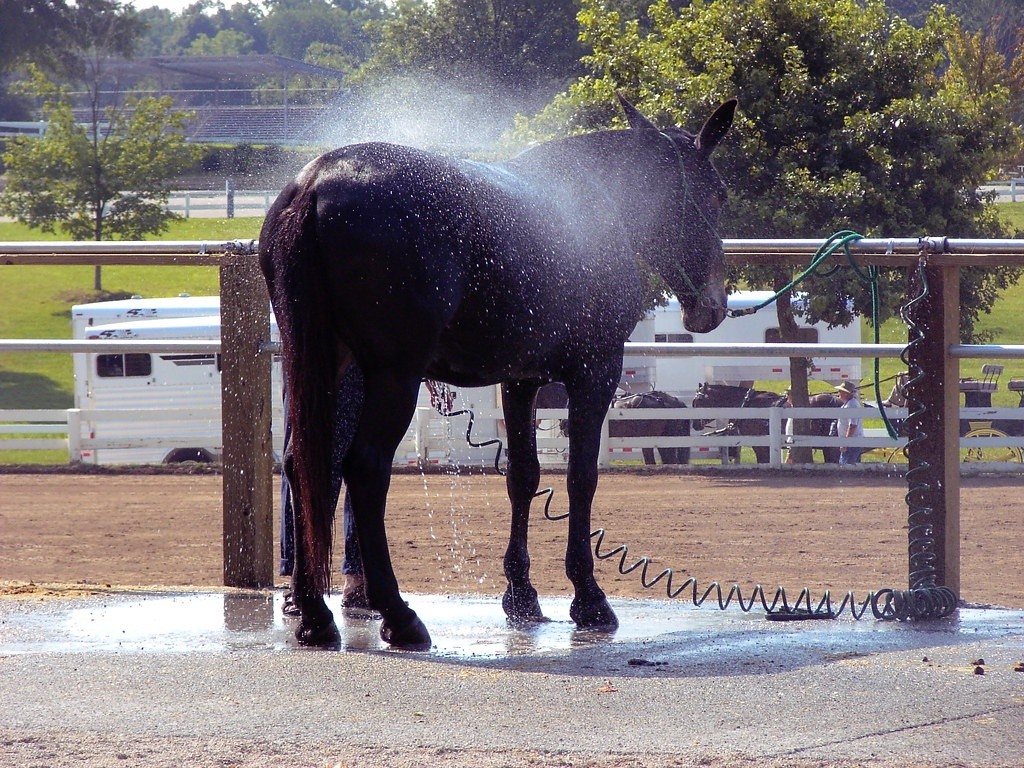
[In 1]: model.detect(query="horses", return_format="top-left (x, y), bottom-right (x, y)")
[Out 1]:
top-left (249, 88), bottom-right (739, 653)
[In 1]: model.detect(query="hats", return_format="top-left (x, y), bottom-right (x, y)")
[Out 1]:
top-left (785, 385), bottom-right (791, 391)
top-left (834, 381), bottom-right (858, 396)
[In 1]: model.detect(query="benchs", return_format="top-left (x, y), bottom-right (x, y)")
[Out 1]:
top-left (959, 364), bottom-right (1005, 407)
top-left (1008, 375), bottom-right (1024, 407)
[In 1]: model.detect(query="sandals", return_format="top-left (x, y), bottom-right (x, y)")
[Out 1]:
top-left (340, 594), bottom-right (383, 620)
top-left (281, 587), bottom-right (302, 614)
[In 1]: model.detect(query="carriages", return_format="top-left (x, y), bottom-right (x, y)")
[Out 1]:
top-left (535, 361), bottom-right (1024, 462)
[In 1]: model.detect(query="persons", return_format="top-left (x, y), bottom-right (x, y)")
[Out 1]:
top-left (784, 385), bottom-right (794, 464)
top-left (279, 339), bottom-right (387, 616)
top-left (834, 381), bottom-right (865, 465)
top-left (881, 371), bottom-right (909, 407)
